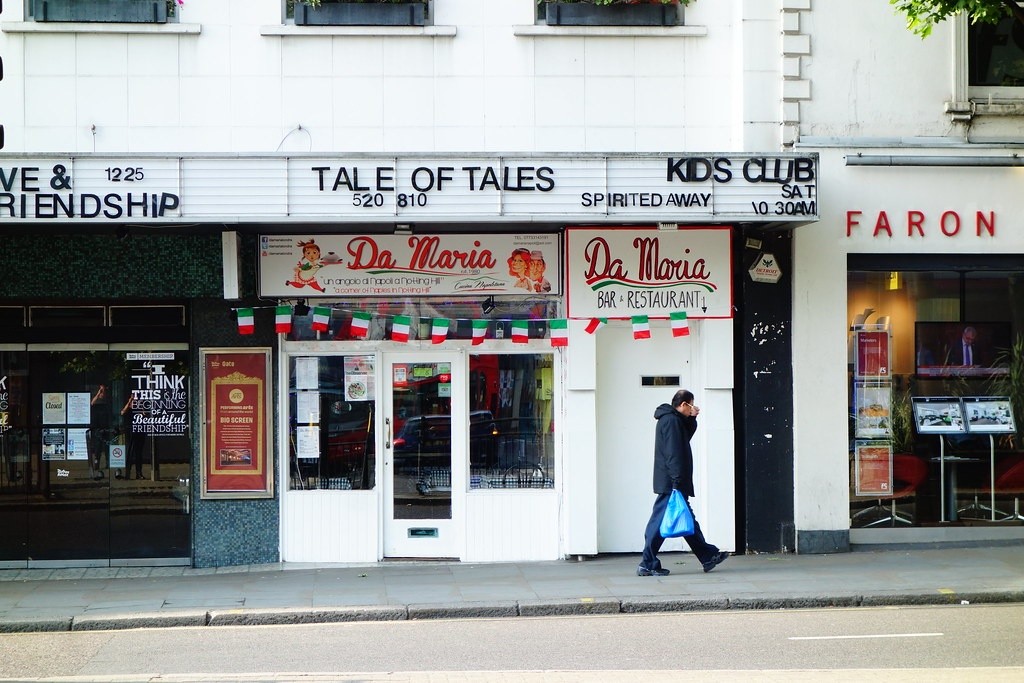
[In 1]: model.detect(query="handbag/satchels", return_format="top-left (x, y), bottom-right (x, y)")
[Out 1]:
top-left (660, 489), bottom-right (694, 538)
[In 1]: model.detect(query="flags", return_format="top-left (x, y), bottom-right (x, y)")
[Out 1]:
top-left (670, 312), bottom-right (689, 337)
top-left (432, 318), bottom-right (450, 344)
top-left (632, 315), bottom-right (650, 339)
top-left (585, 317), bottom-right (607, 335)
top-left (550, 319), bottom-right (568, 346)
top-left (391, 315), bottom-right (411, 342)
top-left (351, 312), bottom-right (370, 336)
top-left (237, 308), bottom-right (254, 334)
top-left (511, 321), bottom-right (528, 343)
top-left (312, 307), bottom-right (331, 331)
top-left (276, 307), bottom-right (291, 333)
top-left (472, 320), bottom-right (487, 345)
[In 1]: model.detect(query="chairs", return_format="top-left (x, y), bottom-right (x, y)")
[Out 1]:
top-left (852, 454), bottom-right (927, 525)
top-left (984, 456), bottom-right (1024, 523)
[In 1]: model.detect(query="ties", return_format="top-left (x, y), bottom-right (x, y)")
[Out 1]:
top-left (965, 344), bottom-right (971, 367)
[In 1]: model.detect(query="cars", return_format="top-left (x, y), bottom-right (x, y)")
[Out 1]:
top-left (393, 411), bottom-right (499, 476)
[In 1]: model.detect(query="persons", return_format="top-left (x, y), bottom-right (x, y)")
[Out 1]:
top-left (917, 336), bottom-right (938, 367)
top-left (636, 390), bottom-right (730, 577)
top-left (120, 394), bottom-right (148, 479)
top-left (949, 326), bottom-right (981, 367)
top-left (90, 385), bottom-right (122, 480)
top-left (943, 412), bottom-right (951, 425)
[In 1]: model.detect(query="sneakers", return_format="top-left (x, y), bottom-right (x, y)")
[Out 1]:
top-left (703, 551), bottom-right (729, 572)
top-left (637, 565), bottom-right (670, 576)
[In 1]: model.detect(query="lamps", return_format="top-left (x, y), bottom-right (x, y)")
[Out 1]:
top-left (482, 296), bottom-right (496, 315)
top-left (294, 300), bottom-right (310, 316)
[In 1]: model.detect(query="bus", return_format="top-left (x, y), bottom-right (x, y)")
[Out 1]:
top-left (290, 373), bottom-right (370, 476)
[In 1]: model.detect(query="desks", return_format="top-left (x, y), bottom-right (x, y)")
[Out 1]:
top-left (929, 457), bottom-right (981, 522)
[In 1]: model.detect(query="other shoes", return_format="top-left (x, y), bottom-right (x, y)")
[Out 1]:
top-left (125, 476), bottom-right (130, 481)
top-left (136, 474), bottom-right (144, 481)
